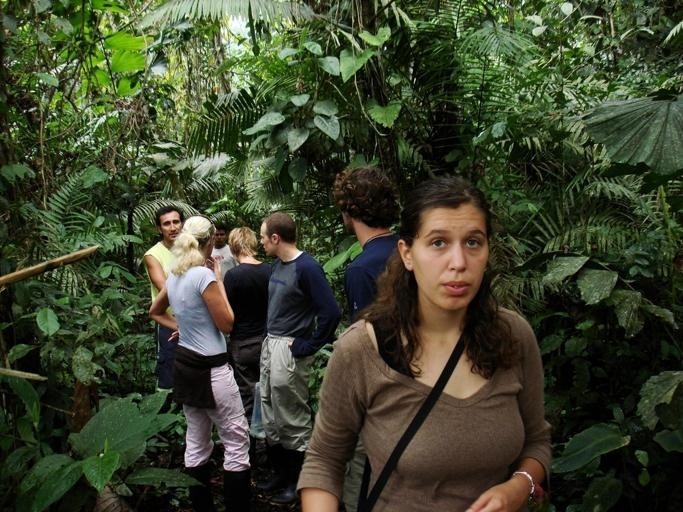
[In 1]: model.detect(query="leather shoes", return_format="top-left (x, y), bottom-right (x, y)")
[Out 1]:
top-left (256, 478), bottom-right (287, 491)
top-left (269, 484), bottom-right (297, 504)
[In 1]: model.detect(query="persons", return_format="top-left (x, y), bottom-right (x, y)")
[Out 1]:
top-left (222, 226), bottom-right (274, 474)
top-left (254, 210), bottom-right (342, 507)
top-left (331, 165), bottom-right (403, 512)
top-left (293, 175), bottom-right (554, 512)
top-left (148, 210), bottom-right (255, 512)
top-left (210, 222), bottom-right (240, 284)
top-left (141, 204), bottom-right (186, 454)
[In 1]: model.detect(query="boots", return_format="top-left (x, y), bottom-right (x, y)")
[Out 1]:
top-left (184, 461), bottom-right (217, 512)
top-left (223, 468), bottom-right (270, 512)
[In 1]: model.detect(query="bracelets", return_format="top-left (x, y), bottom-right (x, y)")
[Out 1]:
top-left (509, 471), bottom-right (535, 497)
